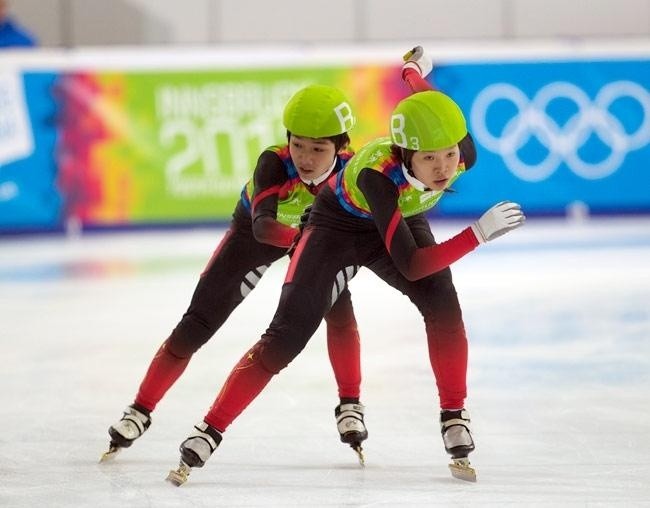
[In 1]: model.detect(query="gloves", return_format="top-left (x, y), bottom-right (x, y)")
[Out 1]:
top-left (400, 45), bottom-right (435, 80)
top-left (469, 200), bottom-right (527, 247)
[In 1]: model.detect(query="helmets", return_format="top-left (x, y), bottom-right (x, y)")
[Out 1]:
top-left (282, 84), bottom-right (358, 138)
top-left (390, 90), bottom-right (468, 152)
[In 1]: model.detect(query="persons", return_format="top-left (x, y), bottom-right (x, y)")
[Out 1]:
top-left (105, 81), bottom-right (368, 444)
top-left (177, 45), bottom-right (526, 466)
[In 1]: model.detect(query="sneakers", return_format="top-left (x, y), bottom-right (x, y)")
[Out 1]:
top-left (334, 401), bottom-right (368, 443)
top-left (108, 403), bottom-right (153, 448)
top-left (179, 421), bottom-right (224, 469)
top-left (438, 407), bottom-right (476, 456)
